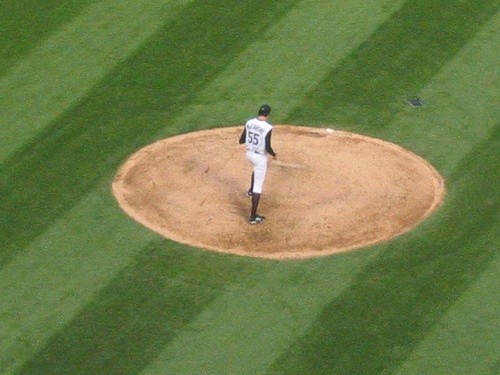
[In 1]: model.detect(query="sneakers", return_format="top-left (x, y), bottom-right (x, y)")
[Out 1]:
top-left (250, 214), bottom-right (266, 225)
top-left (247, 187), bottom-right (253, 196)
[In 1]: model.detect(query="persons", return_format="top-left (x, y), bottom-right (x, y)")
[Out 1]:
top-left (239, 103), bottom-right (278, 225)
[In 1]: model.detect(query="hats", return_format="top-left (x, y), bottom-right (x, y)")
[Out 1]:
top-left (258, 104), bottom-right (273, 118)
